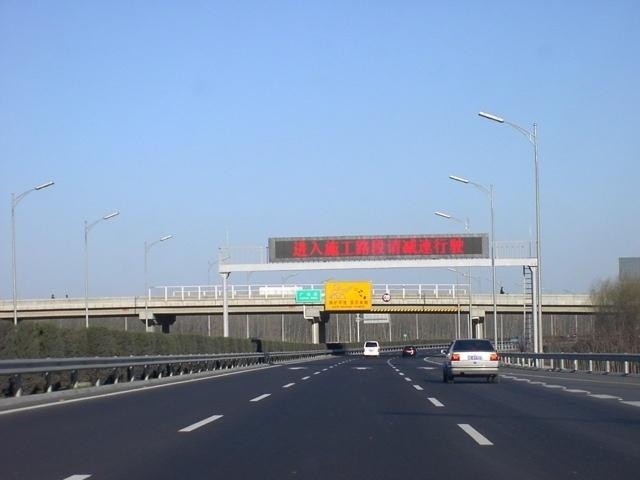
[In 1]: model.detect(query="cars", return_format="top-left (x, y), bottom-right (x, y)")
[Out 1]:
top-left (440, 339), bottom-right (499, 383)
top-left (363, 341), bottom-right (380, 360)
top-left (402, 346), bottom-right (416, 359)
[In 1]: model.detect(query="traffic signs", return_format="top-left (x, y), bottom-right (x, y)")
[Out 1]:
top-left (295, 289), bottom-right (321, 303)
top-left (325, 281), bottom-right (371, 312)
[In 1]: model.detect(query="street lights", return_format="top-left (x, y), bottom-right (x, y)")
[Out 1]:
top-left (435, 212), bottom-right (473, 340)
top-left (11, 181), bottom-right (55, 325)
top-left (448, 267), bottom-right (472, 339)
top-left (448, 175), bottom-right (497, 352)
top-left (144, 234), bottom-right (173, 334)
top-left (84, 211), bottom-right (120, 329)
top-left (478, 110), bottom-right (544, 366)
